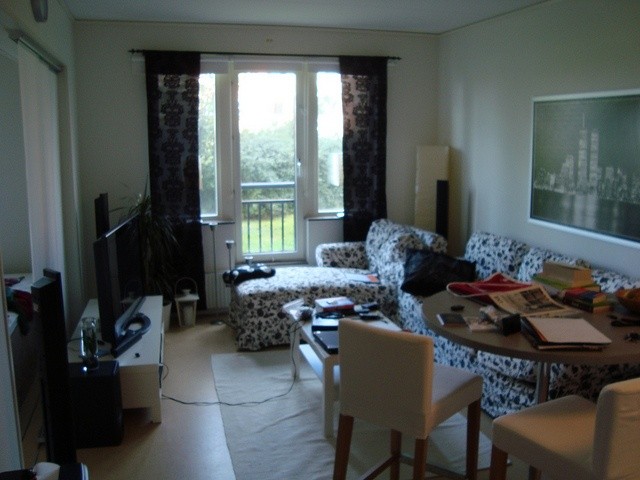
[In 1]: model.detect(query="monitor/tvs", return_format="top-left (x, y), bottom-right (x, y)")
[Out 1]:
top-left (94, 211), bottom-right (151, 343)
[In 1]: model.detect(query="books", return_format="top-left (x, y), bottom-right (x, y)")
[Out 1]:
top-left (489, 259), bottom-right (617, 354)
top-left (314, 296), bottom-right (355, 313)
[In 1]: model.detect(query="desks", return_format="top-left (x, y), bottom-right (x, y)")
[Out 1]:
top-left (422, 292), bottom-right (640, 479)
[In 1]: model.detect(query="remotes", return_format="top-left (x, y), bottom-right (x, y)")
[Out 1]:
top-left (362, 304), bottom-right (380, 310)
top-left (360, 314), bottom-right (381, 319)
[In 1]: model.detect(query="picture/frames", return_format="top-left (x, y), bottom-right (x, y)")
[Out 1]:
top-left (526, 89), bottom-right (639, 250)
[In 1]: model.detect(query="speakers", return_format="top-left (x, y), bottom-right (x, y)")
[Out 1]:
top-left (95, 192), bottom-right (110, 240)
top-left (68, 360), bottom-right (125, 449)
top-left (31, 268), bottom-right (75, 461)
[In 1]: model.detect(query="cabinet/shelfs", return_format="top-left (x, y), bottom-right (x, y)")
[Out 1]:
top-left (67, 295), bottom-right (164, 425)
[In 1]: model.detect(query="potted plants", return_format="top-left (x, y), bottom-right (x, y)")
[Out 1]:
top-left (105, 172), bottom-right (184, 333)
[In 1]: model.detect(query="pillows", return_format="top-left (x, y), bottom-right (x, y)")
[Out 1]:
top-left (401, 248), bottom-right (476, 296)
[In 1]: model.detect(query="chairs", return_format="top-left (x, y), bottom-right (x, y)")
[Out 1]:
top-left (489, 380), bottom-right (639, 478)
top-left (331, 320), bottom-right (483, 479)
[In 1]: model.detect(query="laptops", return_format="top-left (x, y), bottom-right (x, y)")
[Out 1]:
top-left (313, 331), bottom-right (339, 354)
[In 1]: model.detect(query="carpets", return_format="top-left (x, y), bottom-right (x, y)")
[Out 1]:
top-left (211, 351), bottom-right (512, 480)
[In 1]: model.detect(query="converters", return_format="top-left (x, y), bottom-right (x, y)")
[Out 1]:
top-left (302, 310), bottom-right (312, 321)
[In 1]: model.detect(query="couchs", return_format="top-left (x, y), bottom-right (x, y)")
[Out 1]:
top-left (393, 233), bottom-right (640, 415)
top-left (228, 218), bottom-right (446, 351)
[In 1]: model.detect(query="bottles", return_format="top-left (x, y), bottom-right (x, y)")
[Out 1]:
top-left (80, 317), bottom-right (100, 371)
